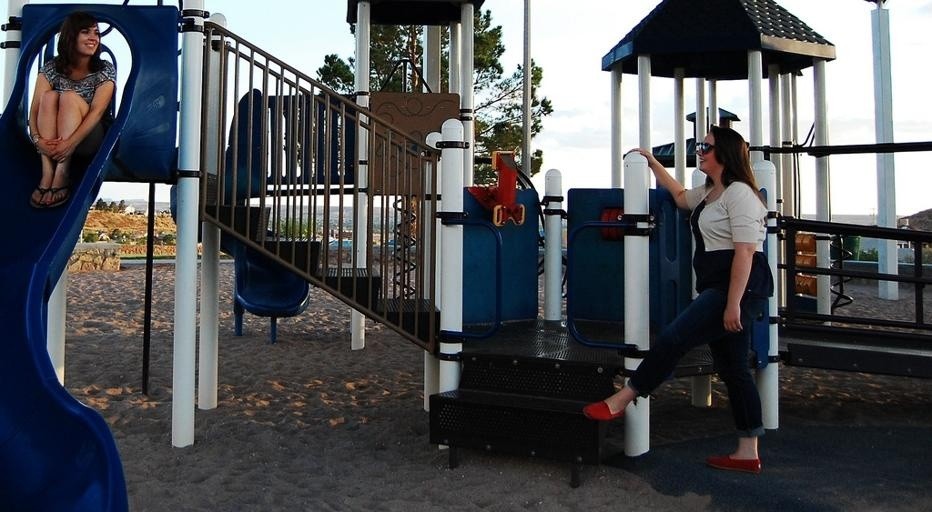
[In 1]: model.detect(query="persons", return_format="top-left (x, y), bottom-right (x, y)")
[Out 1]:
top-left (26, 11), bottom-right (117, 210)
top-left (579, 122), bottom-right (777, 474)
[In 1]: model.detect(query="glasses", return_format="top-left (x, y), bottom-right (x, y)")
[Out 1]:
top-left (694, 141), bottom-right (715, 154)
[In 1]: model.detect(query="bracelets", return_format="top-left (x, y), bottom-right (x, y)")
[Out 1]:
top-left (32, 134), bottom-right (40, 145)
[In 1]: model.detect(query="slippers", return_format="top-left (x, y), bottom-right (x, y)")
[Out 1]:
top-left (29, 186), bottom-right (49, 208)
top-left (44, 184), bottom-right (71, 209)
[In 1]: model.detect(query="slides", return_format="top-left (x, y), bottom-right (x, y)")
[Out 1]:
top-left (172, 149), bottom-right (310, 318)
top-left (0, 3), bottom-right (177, 511)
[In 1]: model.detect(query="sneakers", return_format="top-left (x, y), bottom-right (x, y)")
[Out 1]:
top-left (582, 399), bottom-right (624, 421)
top-left (704, 452), bottom-right (761, 473)
